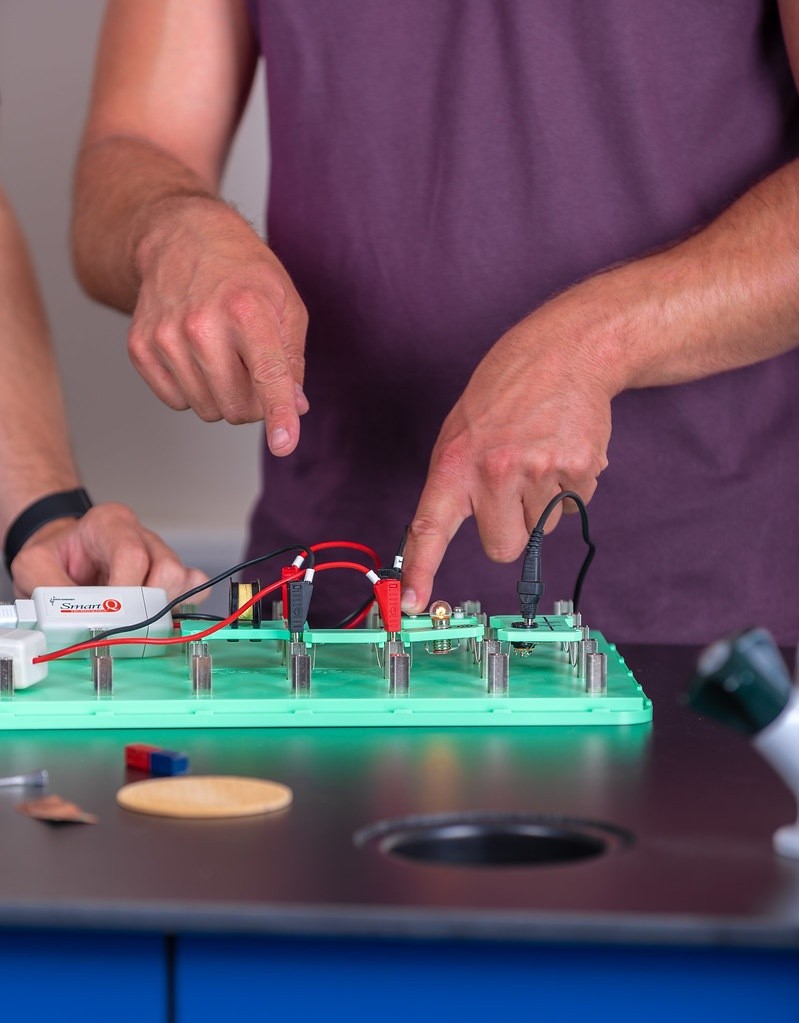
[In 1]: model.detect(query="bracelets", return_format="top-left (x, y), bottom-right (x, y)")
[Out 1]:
top-left (3, 487), bottom-right (92, 580)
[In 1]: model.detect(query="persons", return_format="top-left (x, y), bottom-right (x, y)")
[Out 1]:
top-left (0, 185), bottom-right (210, 613)
top-left (70, 0), bottom-right (798, 646)
